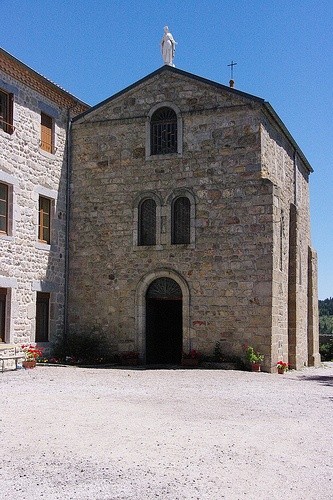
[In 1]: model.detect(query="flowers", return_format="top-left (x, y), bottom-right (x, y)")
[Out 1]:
top-left (183, 350), bottom-right (204, 361)
top-left (277, 361), bottom-right (288, 370)
top-left (21, 344), bottom-right (44, 362)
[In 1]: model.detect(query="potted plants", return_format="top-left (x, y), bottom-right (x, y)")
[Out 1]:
top-left (245, 344), bottom-right (264, 372)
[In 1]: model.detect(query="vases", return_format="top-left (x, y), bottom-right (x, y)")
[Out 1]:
top-left (22, 361), bottom-right (36, 368)
top-left (182, 359), bottom-right (199, 365)
top-left (278, 368), bottom-right (284, 374)
